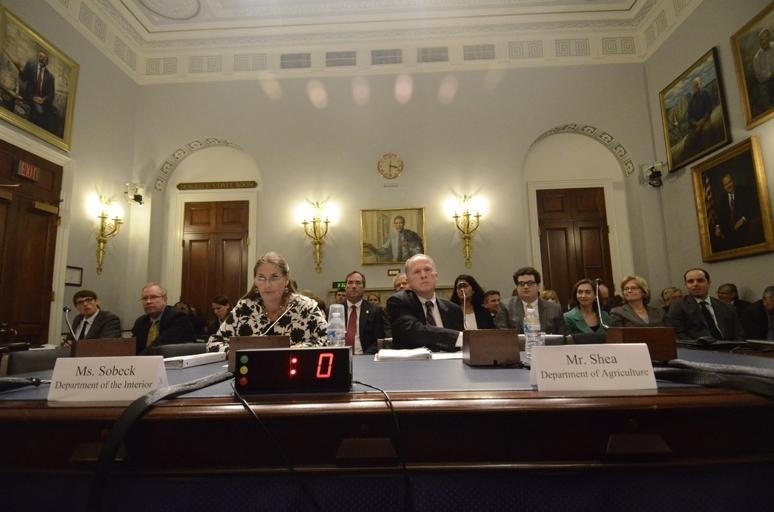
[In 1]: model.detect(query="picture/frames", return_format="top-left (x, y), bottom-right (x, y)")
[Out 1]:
top-left (359, 205), bottom-right (425, 269)
top-left (656, 45), bottom-right (734, 177)
top-left (689, 133), bottom-right (774, 265)
top-left (729, 3), bottom-right (774, 133)
top-left (0, 3), bottom-right (81, 155)
top-left (63, 264), bottom-right (83, 285)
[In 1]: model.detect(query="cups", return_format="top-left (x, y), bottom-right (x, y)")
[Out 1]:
top-left (326, 303), bottom-right (347, 333)
top-left (523, 309), bottom-right (540, 330)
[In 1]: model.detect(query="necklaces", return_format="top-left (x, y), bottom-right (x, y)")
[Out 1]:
top-left (261, 301), bottom-right (286, 324)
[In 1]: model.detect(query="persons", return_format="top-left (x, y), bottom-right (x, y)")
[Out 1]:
top-left (212, 298), bottom-right (233, 329)
top-left (752, 28), bottom-right (774, 108)
top-left (363, 216), bottom-right (423, 262)
top-left (657, 269), bottom-right (771, 340)
top-left (449, 267), bottom-right (667, 333)
top-left (19, 50), bottom-right (55, 129)
top-left (687, 76), bottom-right (717, 146)
top-left (714, 171), bottom-right (753, 249)
top-left (393, 273), bottom-right (410, 292)
top-left (386, 254), bottom-right (466, 353)
top-left (130, 282), bottom-right (216, 355)
top-left (63, 290), bottom-right (122, 351)
top-left (205, 251), bottom-right (330, 357)
top-left (334, 271), bottom-right (391, 355)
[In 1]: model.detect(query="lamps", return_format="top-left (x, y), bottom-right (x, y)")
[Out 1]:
top-left (85, 191), bottom-right (124, 276)
top-left (297, 198), bottom-right (338, 275)
top-left (449, 196), bottom-right (488, 264)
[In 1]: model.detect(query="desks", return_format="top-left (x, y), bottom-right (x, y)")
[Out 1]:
top-left (0, 340), bottom-right (774, 512)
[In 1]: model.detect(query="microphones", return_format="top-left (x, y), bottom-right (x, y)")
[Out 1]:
top-left (261, 300), bottom-right (297, 335)
top-left (594, 277), bottom-right (607, 330)
top-left (459, 282), bottom-right (468, 329)
top-left (63, 304), bottom-right (76, 340)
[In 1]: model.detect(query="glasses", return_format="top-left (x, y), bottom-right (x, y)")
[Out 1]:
top-left (77, 297), bottom-right (93, 305)
top-left (138, 295), bottom-right (162, 303)
top-left (518, 281), bottom-right (536, 286)
top-left (255, 274), bottom-right (285, 282)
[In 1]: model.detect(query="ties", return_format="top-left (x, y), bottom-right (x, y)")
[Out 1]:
top-left (78, 321), bottom-right (88, 340)
top-left (699, 301), bottom-right (723, 341)
top-left (148, 320), bottom-right (157, 344)
top-left (346, 305), bottom-right (357, 355)
top-left (425, 301), bottom-right (436, 326)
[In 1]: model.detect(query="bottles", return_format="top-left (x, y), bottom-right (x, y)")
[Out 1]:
top-left (327, 312), bottom-right (346, 346)
top-left (523, 330), bottom-right (540, 359)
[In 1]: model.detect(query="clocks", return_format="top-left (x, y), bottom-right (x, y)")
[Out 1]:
top-left (375, 151), bottom-right (403, 181)
